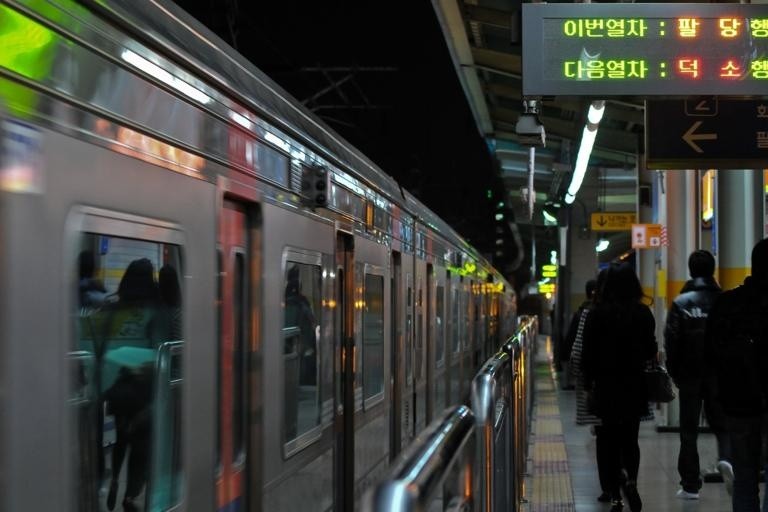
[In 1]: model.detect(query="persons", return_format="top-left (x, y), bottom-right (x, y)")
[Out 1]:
top-left (282, 265), bottom-right (316, 355)
top-left (88, 255), bottom-right (173, 512)
top-left (155, 264), bottom-right (183, 371)
top-left (700, 235), bottom-right (767, 511)
top-left (75, 249), bottom-right (112, 489)
top-left (558, 262), bottom-right (661, 512)
top-left (662, 248), bottom-right (734, 503)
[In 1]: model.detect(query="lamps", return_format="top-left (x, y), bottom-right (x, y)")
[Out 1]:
top-left (561, 99), bottom-right (610, 205)
top-left (542, 187), bottom-right (591, 241)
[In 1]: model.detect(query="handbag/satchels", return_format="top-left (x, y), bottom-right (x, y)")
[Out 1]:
top-left (642, 360), bottom-right (676, 403)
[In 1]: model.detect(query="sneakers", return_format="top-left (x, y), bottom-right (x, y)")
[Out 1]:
top-left (676, 489), bottom-right (701, 500)
top-left (597, 483), bottom-right (642, 512)
top-left (716, 457), bottom-right (735, 497)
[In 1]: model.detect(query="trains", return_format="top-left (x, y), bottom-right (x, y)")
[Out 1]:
top-left (0, 3), bottom-right (524, 511)
